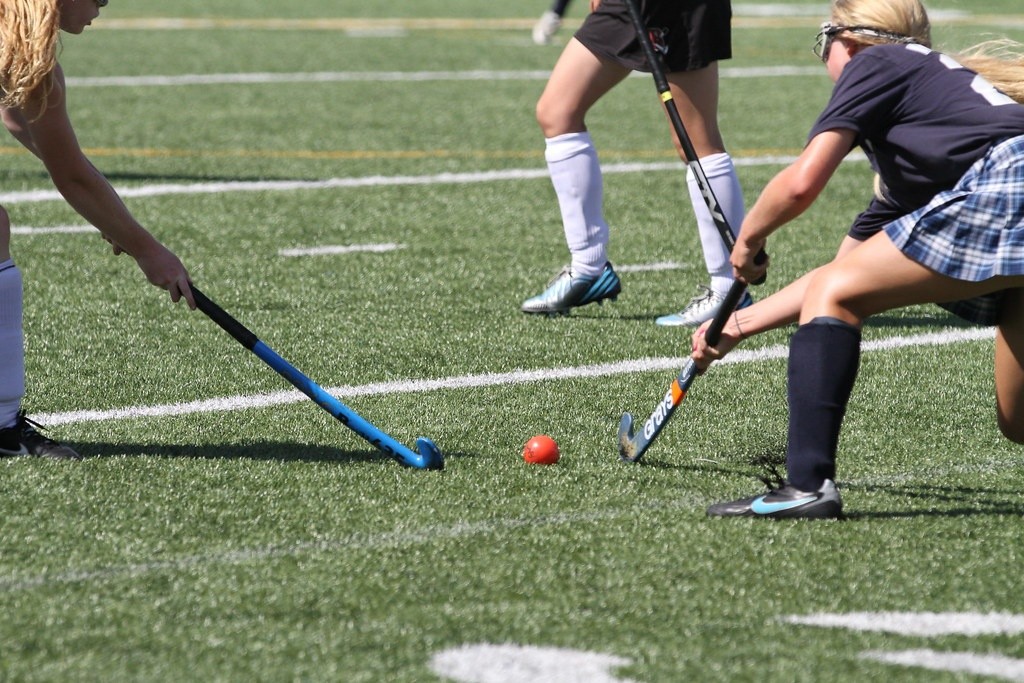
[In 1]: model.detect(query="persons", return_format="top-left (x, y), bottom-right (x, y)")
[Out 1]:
top-left (692, 0), bottom-right (1024, 518)
top-left (522, 0), bottom-right (754, 326)
top-left (0, 0), bottom-right (196, 459)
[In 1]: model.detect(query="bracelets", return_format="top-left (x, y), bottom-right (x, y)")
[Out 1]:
top-left (734, 312), bottom-right (746, 339)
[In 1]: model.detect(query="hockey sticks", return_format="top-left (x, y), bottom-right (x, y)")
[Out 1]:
top-left (193, 282), bottom-right (446, 474)
top-left (619, 247), bottom-right (770, 462)
top-left (626, 1), bottom-right (739, 253)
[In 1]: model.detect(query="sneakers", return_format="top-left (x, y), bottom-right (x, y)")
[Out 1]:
top-left (521, 261), bottom-right (622, 314)
top-left (656, 285), bottom-right (753, 326)
top-left (0, 408), bottom-right (82, 462)
top-left (533, 10), bottom-right (560, 45)
top-left (706, 456), bottom-right (843, 518)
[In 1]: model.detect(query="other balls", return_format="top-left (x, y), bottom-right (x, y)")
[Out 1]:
top-left (524, 435), bottom-right (559, 463)
